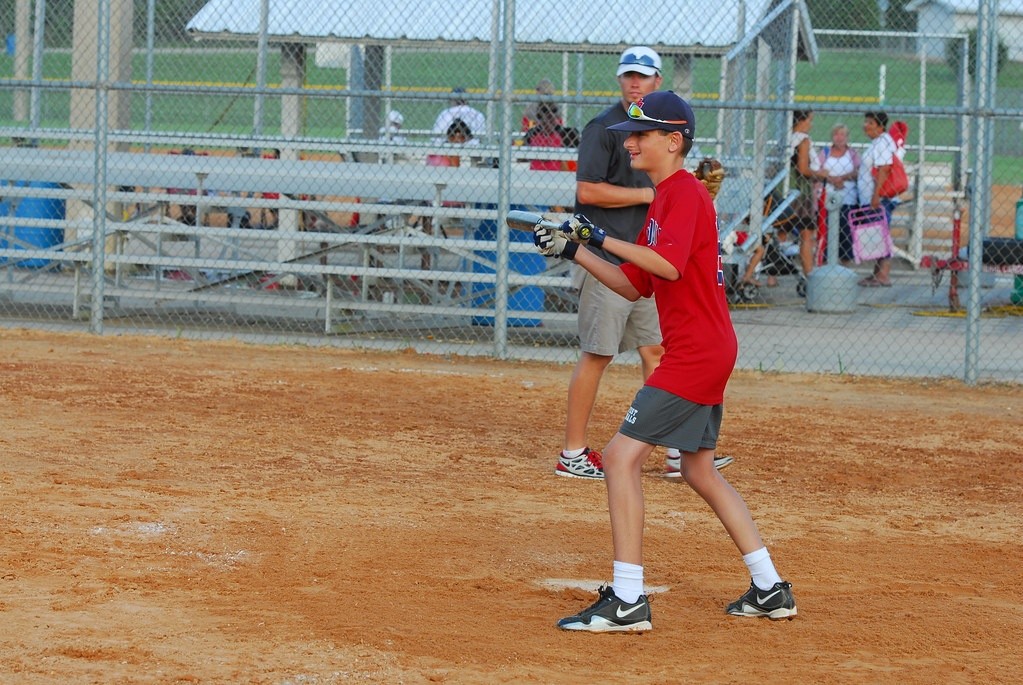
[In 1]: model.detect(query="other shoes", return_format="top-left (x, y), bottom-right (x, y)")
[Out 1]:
top-left (858, 274), bottom-right (892, 287)
top-left (796, 280), bottom-right (806, 296)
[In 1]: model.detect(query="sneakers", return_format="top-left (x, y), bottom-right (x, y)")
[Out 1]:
top-left (725, 577), bottom-right (798, 618)
top-left (662, 454), bottom-right (734, 483)
top-left (555, 445), bottom-right (605, 479)
top-left (557, 581), bottom-right (652, 632)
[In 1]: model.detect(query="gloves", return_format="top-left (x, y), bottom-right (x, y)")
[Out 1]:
top-left (558, 214), bottom-right (607, 250)
top-left (532, 213), bottom-right (581, 261)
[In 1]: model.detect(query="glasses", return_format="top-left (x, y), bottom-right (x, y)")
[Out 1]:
top-left (618, 53), bottom-right (662, 73)
top-left (627, 102), bottom-right (687, 124)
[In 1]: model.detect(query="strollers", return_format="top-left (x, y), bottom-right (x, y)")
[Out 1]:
top-left (730, 194), bottom-right (809, 301)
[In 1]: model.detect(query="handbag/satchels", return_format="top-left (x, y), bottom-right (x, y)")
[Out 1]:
top-left (871, 136), bottom-right (908, 197)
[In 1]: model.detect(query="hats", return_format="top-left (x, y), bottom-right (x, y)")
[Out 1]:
top-left (616, 46), bottom-right (662, 77)
top-left (604, 90), bottom-right (695, 139)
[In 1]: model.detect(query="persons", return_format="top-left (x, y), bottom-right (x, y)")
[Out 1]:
top-left (427, 87), bottom-right (494, 169)
top-left (553, 47), bottom-right (737, 480)
top-left (716, 109), bottom-right (908, 286)
top-left (446, 119), bottom-right (484, 169)
top-left (534, 92), bottom-right (798, 634)
top-left (510, 78), bottom-right (578, 171)
top-left (380, 111), bottom-right (424, 229)
